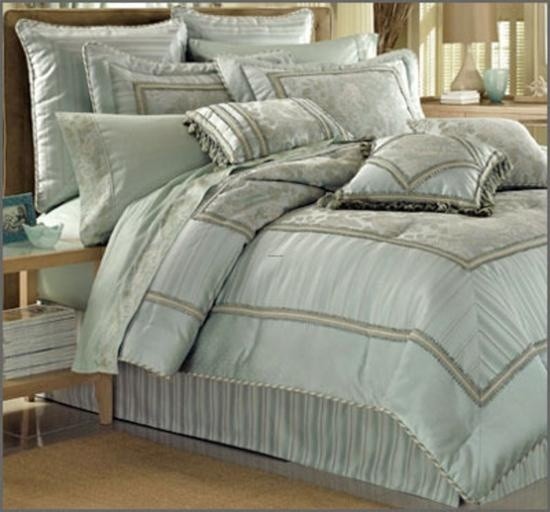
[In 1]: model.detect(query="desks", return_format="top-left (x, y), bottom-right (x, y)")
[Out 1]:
top-left (422, 99), bottom-right (548, 133)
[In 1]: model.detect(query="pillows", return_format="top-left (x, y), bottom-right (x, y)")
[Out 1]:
top-left (317, 130), bottom-right (516, 216)
top-left (53, 109), bottom-right (206, 248)
top-left (189, 30), bottom-right (381, 69)
top-left (353, 46), bottom-right (427, 118)
top-left (211, 48), bottom-right (295, 101)
top-left (240, 61), bottom-right (420, 143)
top-left (11, 14), bottom-right (189, 211)
top-left (77, 39), bottom-right (233, 117)
top-left (169, 5), bottom-right (316, 47)
top-left (183, 93), bottom-right (355, 168)
top-left (406, 115), bottom-right (548, 189)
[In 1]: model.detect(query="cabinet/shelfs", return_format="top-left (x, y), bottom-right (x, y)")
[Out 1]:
top-left (2, 242), bottom-right (116, 450)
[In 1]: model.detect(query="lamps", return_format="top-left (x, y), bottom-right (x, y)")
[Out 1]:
top-left (440, 3), bottom-right (507, 93)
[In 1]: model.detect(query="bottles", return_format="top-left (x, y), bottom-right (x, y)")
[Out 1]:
top-left (450, 38), bottom-right (485, 95)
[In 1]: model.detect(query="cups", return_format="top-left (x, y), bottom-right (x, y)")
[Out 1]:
top-left (486, 68), bottom-right (508, 104)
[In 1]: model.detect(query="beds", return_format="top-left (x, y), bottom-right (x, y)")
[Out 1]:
top-left (1, 2), bottom-right (548, 511)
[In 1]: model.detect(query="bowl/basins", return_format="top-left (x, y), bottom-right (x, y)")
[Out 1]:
top-left (22, 223), bottom-right (63, 249)
